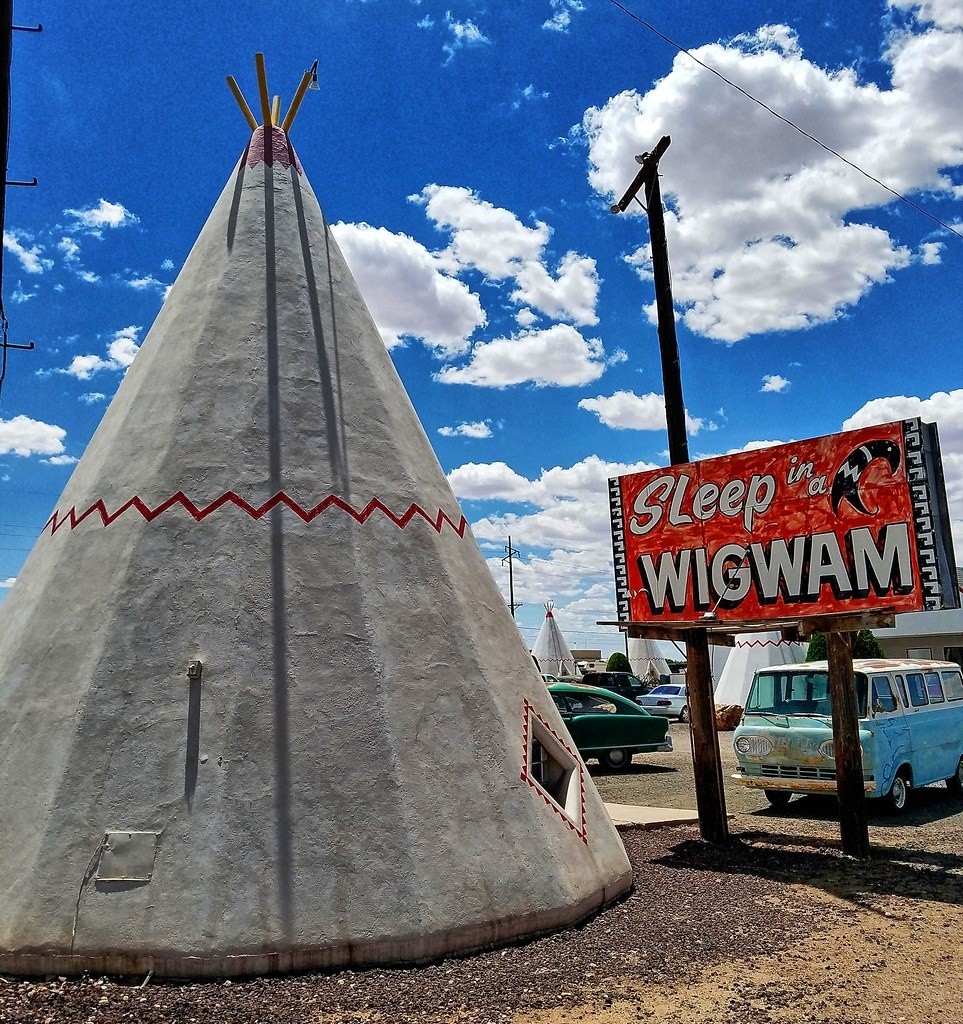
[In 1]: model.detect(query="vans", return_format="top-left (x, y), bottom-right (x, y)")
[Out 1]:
top-left (731, 658), bottom-right (963, 814)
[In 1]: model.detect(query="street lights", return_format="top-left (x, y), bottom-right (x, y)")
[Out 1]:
top-left (573, 660), bottom-right (578, 676)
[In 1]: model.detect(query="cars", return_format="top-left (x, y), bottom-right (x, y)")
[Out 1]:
top-left (636, 684), bottom-right (691, 724)
top-left (540, 674), bottom-right (559, 684)
top-left (581, 672), bottom-right (648, 704)
top-left (542, 682), bottom-right (673, 772)
